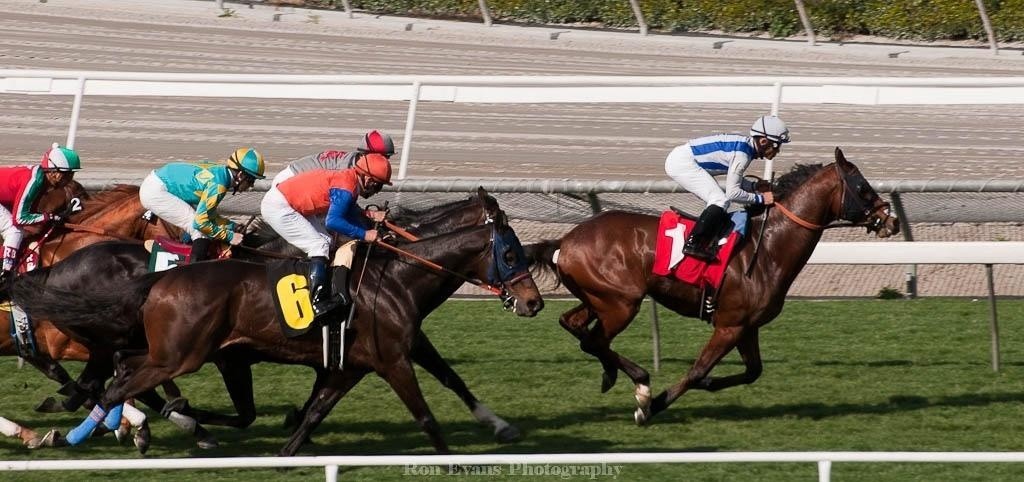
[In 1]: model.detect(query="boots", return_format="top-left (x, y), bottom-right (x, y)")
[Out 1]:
top-left (308, 258), bottom-right (340, 316)
top-left (682, 204), bottom-right (724, 258)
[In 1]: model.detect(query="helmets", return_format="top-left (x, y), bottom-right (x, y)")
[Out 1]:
top-left (750, 116), bottom-right (791, 144)
top-left (227, 148), bottom-right (266, 180)
top-left (354, 129), bottom-right (394, 185)
top-left (42, 146), bottom-right (82, 172)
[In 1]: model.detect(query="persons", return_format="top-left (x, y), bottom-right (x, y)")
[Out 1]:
top-left (0, 142), bottom-right (79, 299)
top-left (270, 128), bottom-right (399, 192)
top-left (254, 151), bottom-right (394, 318)
top-left (664, 114), bottom-right (792, 263)
top-left (139, 146), bottom-right (267, 264)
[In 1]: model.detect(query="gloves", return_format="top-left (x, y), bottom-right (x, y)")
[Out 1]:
top-left (45, 212), bottom-right (67, 226)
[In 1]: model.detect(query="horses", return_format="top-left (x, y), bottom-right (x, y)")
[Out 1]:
top-left (522, 145), bottom-right (901, 428)
top-left (0, 177), bottom-right (545, 476)
